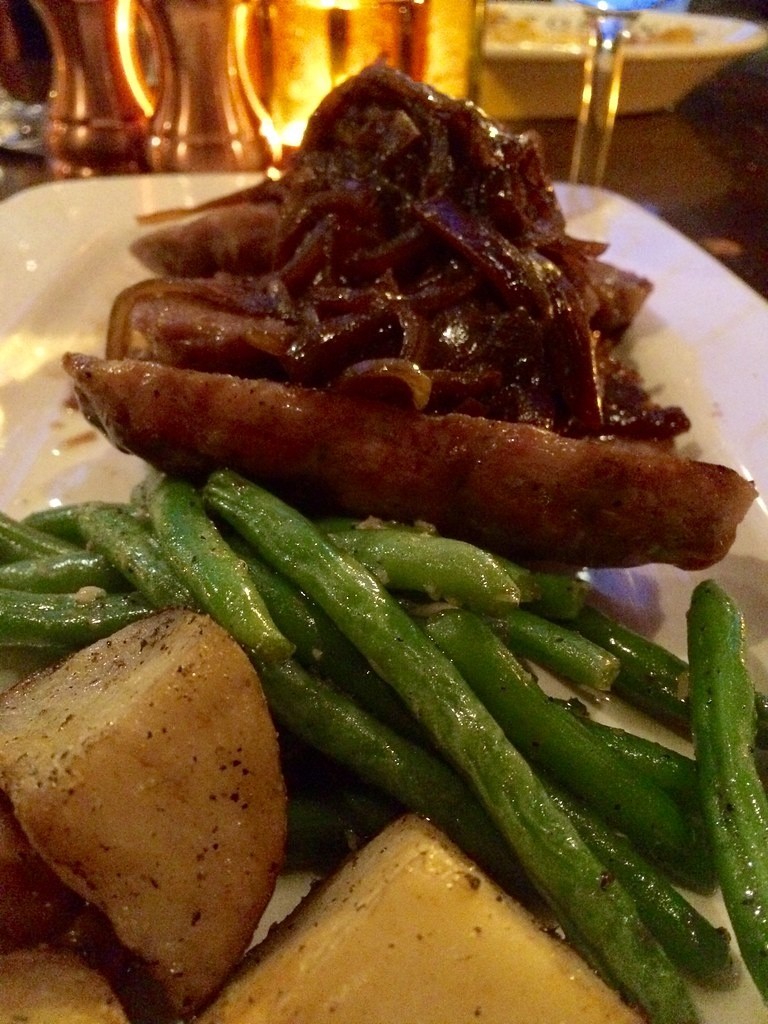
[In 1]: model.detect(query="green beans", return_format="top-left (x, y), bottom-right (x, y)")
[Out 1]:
top-left (0, 468), bottom-right (768, 1024)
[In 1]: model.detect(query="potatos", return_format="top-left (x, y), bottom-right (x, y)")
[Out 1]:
top-left (0, 944), bottom-right (131, 1024)
top-left (0, 798), bottom-right (90, 955)
top-left (0, 607), bottom-right (288, 1020)
top-left (178, 815), bottom-right (651, 1024)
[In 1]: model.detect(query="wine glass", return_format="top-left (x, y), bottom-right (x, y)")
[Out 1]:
top-left (555, 0), bottom-right (688, 188)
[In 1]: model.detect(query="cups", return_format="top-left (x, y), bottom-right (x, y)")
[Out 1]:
top-left (230, 0), bottom-right (432, 180)
top-left (116, 0), bottom-right (270, 175)
top-left (0, 0), bottom-right (153, 176)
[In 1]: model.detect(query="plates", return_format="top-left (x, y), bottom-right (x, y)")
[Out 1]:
top-left (0, 172), bottom-right (768, 1024)
top-left (476, 2), bottom-right (768, 119)
